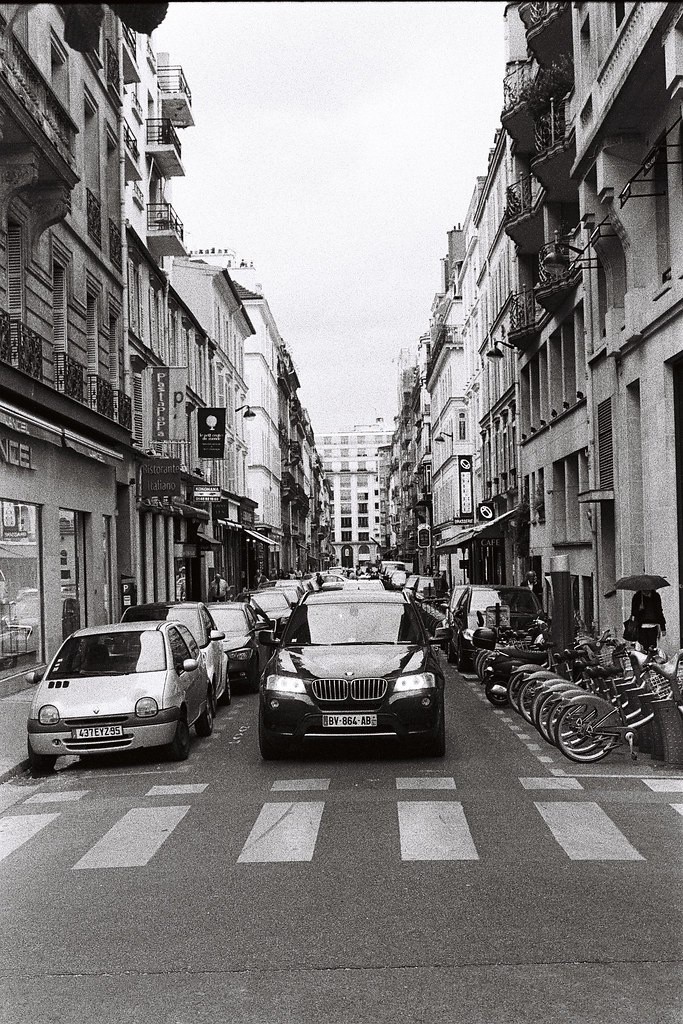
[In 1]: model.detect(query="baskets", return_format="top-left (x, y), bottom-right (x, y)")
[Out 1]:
top-left (595, 646), bottom-right (616, 666)
top-left (640, 661), bottom-right (683, 699)
top-left (618, 652), bottom-right (653, 678)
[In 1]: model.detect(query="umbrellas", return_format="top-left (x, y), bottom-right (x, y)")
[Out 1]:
top-left (612, 574), bottom-right (671, 606)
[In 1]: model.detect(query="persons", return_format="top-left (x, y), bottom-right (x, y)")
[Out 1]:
top-left (519, 571), bottom-right (543, 603)
top-left (177, 567), bottom-right (185, 601)
top-left (622, 591), bottom-right (667, 653)
top-left (253, 558), bottom-right (380, 592)
top-left (210, 573), bottom-right (234, 602)
top-left (426, 565), bottom-right (433, 576)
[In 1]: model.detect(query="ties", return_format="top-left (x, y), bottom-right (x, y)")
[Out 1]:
top-left (217, 582), bottom-right (220, 597)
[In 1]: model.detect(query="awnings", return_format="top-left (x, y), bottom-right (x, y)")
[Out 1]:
top-left (244, 529), bottom-right (280, 546)
top-left (297, 544), bottom-right (308, 551)
top-left (433, 507), bottom-right (515, 555)
top-left (310, 556), bottom-right (318, 560)
top-left (197, 533), bottom-right (221, 544)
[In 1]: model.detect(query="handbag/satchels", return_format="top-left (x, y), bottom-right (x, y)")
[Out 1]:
top-left (623, 609), bottom-right (642, 641)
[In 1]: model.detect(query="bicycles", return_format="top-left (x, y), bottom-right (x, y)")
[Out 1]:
top-left (474, 614), bottom-right (683, 763)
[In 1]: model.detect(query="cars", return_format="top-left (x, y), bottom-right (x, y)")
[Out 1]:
top-left (28, 621), bottom-right (215, 775)
top-left (205, 560), bottom-right (446, 679)
top-left (120, 601), bottom-right (231, 710)
top-left (258, 590), bottom-right (447, 763)
top-left (448, 583), bottom-right (548, 674)
